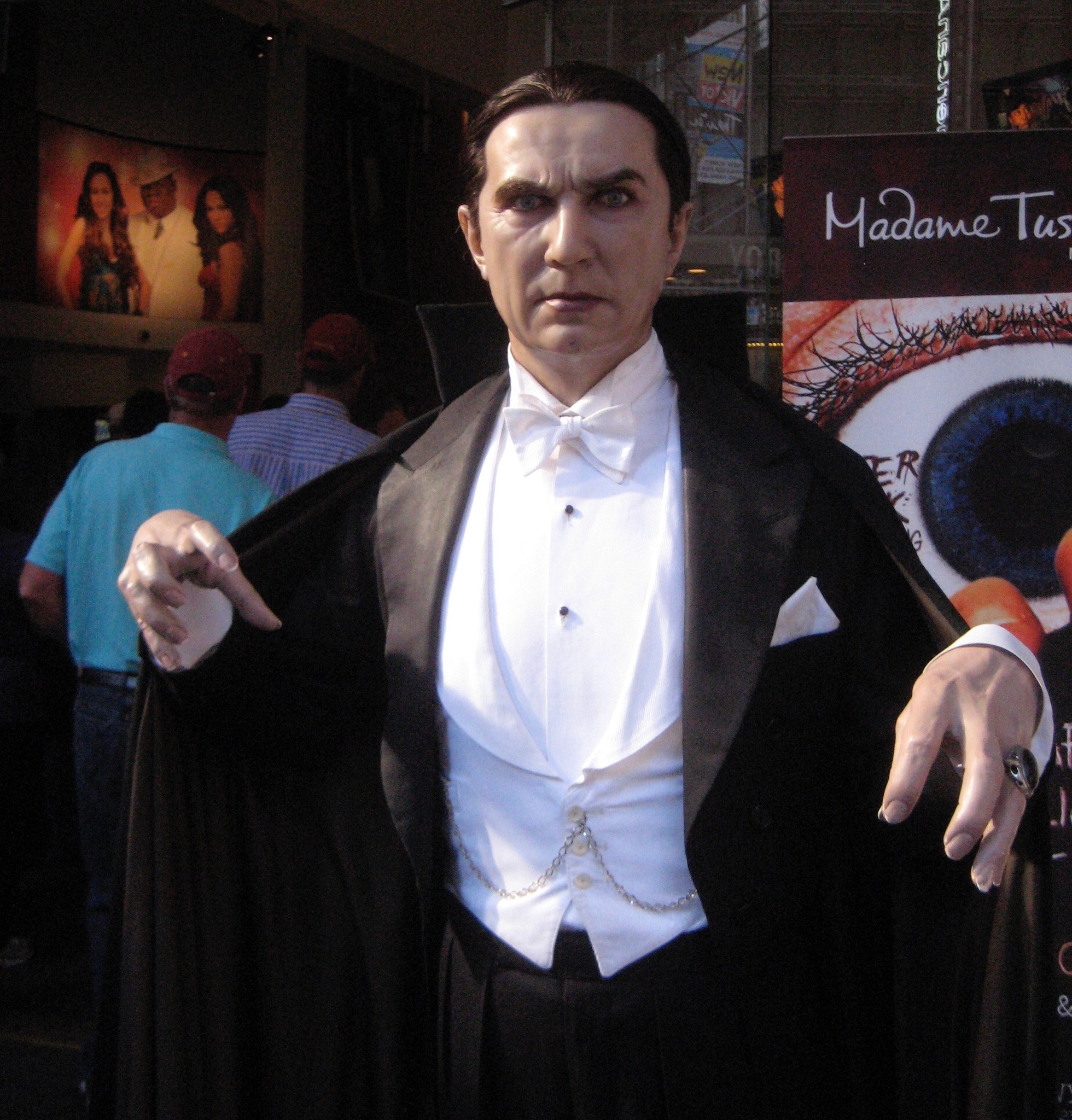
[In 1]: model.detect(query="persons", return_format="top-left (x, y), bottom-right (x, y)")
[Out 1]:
top-left (223, 315), bottom-right (410, 503)
top-left (17, 324), bottom-right (276, 930)
top-left (113, 59), bottom-right (1058, 1120)
top-left (55, 159), bottom-right (262, 322)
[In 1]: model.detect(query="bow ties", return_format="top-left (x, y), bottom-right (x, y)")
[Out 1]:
top-left (503, 394), bottom-right (635, 484)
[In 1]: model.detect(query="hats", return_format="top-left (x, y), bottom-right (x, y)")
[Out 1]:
top-left (130, 153), bottom-right (179, 187)
top-left (300, 312), bottom-right (380, 371)
top-left (166, 326), bottom-right (266, 401)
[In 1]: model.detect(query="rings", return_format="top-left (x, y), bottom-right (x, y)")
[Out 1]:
top-left (1003, 744), bottom-right (1040, 800)
